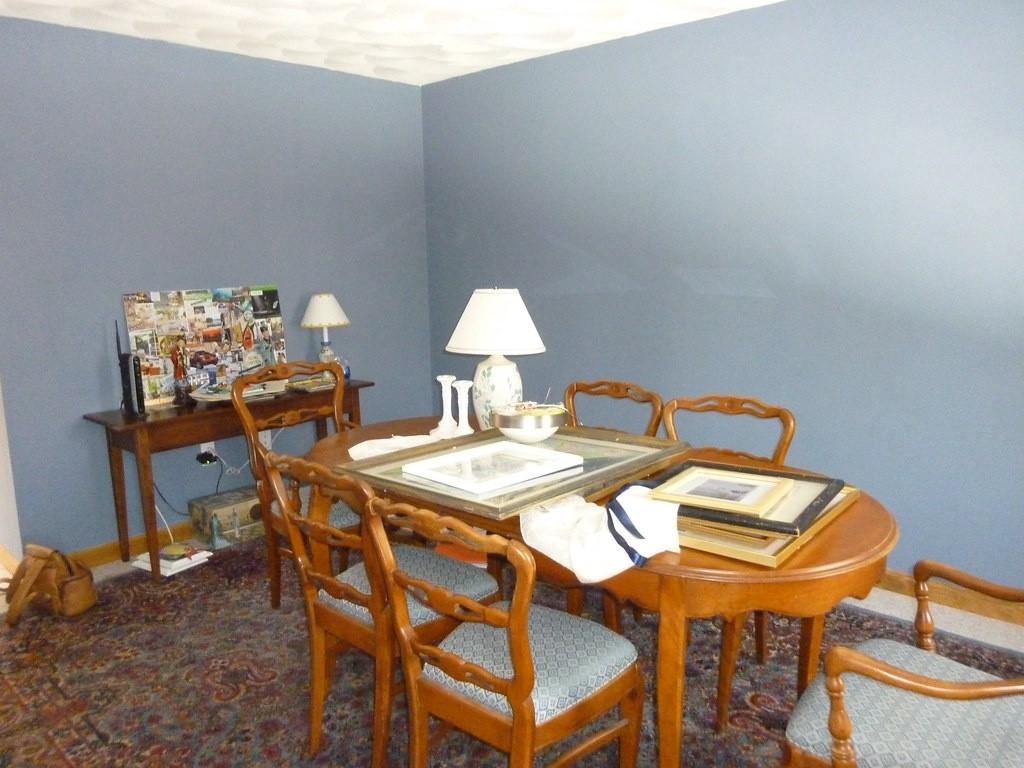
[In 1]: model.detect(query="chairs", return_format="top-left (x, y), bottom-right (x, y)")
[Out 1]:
top-left (230, 361), bottom-right (1024, 768)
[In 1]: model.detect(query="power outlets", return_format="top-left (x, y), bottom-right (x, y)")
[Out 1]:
top-left (200, 441), bottom-right (216, 467)
top-left (258, 429), bottom-right (272, 451)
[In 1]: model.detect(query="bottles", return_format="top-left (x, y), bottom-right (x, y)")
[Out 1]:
top-left (174, 351), bottom-right (191, 405)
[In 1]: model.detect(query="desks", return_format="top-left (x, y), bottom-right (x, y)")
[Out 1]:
top-left (82, 380), bottom-right (375, 581)
top-left (305, 416), bottom-right (900, 767)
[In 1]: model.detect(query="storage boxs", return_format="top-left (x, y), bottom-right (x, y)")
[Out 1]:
top-left (187, 484), bottom-right (262, 536)
top-left (190, 521), bottom-right (265, 544)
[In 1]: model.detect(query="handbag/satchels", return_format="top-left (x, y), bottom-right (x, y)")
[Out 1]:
top-left (5, 544), bottom-right (98, 625)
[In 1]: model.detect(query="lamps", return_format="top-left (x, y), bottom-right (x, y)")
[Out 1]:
top-left (445, 285), bottom-right (546, 431)
top-left (301, 292), bottom-right (350, 381)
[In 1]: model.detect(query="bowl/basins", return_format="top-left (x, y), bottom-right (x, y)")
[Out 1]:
top-left (489, 403), bottom-right (566, 444)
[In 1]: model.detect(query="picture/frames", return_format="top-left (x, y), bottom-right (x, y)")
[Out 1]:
top-left (646, 466), bottom-right (796, 518)
top-left (649, 458), bottom-right (846, 537)
top-left (402, 439), bottom-right (583, 495)
top-left (331, 424), bottom-right (692, 522)
top-left (678, 524), bottom-right (776, 549)
top-left (678, 487), bottom-right (860, 568)
